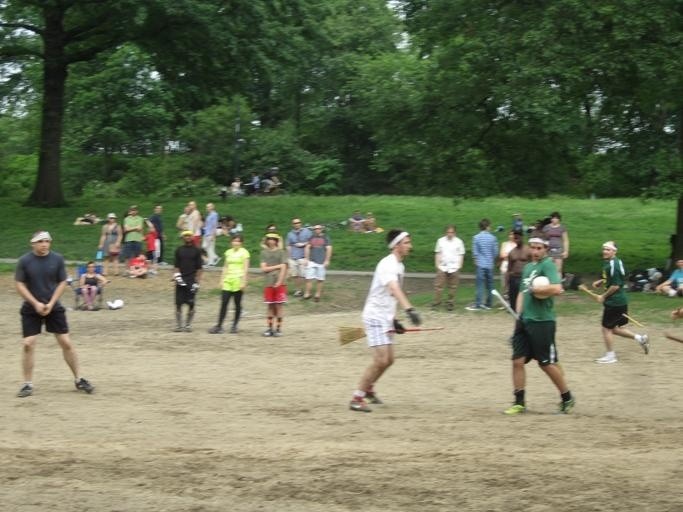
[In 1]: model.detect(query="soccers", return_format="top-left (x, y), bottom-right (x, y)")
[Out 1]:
top-left (531, 276), bottom-right (550, 299)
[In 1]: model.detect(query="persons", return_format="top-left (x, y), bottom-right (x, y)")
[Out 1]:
top-left (14, 231), bottom-right (93, 397)
top-left (348, 229), bottom-right (421, 412)
top-left (505, 229), bottom-right (577, 415)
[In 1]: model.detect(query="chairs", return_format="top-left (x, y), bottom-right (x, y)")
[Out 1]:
top-left (73, 263), bottom-right (106, 312)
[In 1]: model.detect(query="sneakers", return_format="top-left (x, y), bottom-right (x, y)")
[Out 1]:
top-left (349, 400), bottom-right (371, 413)
top-left (17, 384), bottom-right (32, 398)
top-left (640, 336), bottom-right (650, 355)
top-left (595, 352), bottom-right (618, 365)
top-left (85, 268), bottom-right (321, 337)
top-left (432, 300), bottom-right (519, 315)
top-left (503, 404), bottom-right (526, 415)
top-left (366, 392), bottom-right (380, 403)
top-left (76, 379), bottom-right (93, 393)
top-left (561, 398), bottom-right (575, 414)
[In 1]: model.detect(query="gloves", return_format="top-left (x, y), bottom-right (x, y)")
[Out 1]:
top-left (408, 309), bottom-right (421, 325)
top-left (393, 320), bottom-right (405, 334)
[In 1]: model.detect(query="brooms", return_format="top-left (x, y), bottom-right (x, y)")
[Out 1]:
top-left (339, 326), bottom-right (443, 345)
